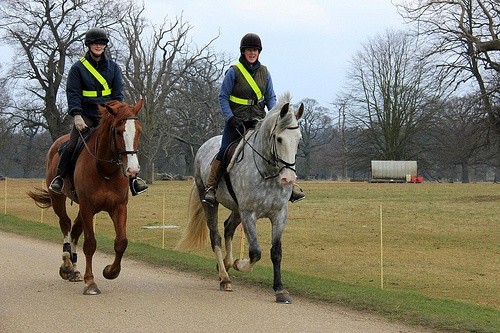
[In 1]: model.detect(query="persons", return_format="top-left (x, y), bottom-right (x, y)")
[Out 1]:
top-left (205, 34), bottom-right (305, 204)
top-left (52, 29), bottom-right (149, 196)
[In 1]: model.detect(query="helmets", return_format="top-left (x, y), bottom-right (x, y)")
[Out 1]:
top-left (240, 33), bottom-right (262, 52)
top-left (85, 28), bottom-right (109, 46)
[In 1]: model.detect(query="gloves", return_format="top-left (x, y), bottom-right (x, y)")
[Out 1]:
top-left (229, 117), bottom-right (243, 128)
top-left (73, 115), bottom-right (86, 131)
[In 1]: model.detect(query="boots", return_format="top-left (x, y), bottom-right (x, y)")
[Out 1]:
top-left (204, 158), bottom-right (222, 203)
top-left (52, 149), bottom-right (73, 191)
top-left (291, 184), bottom-right (305, 200)
top-left (128, 175), bottom-right (148, 196)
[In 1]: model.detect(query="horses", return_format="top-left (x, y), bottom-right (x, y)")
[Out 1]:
top-left (25, 98), bottom-right (145, 296)
top-left (171, 91), bottom-right (304, 304)
